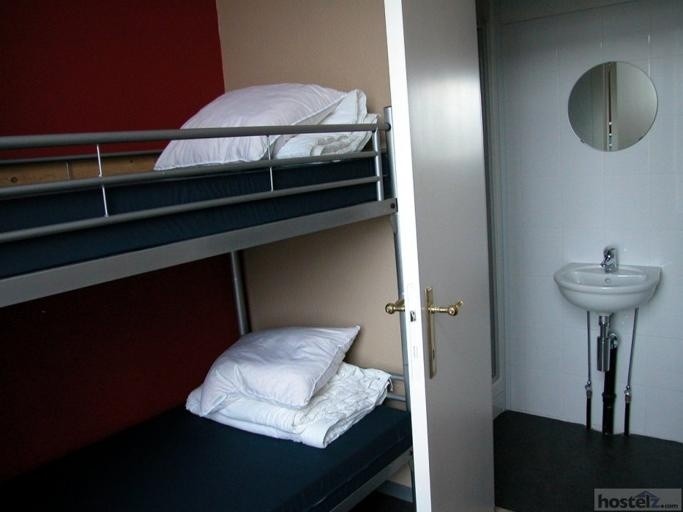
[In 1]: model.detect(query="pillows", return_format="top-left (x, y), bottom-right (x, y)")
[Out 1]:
top-left (152, 85), bottom-right (343, 172)
top-left (201, 326), bottom-right (361, 419)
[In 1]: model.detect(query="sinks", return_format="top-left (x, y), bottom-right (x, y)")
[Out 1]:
top-left (553, 263), bottom-right (661, 316)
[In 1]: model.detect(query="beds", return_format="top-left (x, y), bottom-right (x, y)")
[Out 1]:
top-left (1, 106), bottom-right (415, 511)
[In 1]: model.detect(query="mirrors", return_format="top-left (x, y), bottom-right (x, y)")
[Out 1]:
top-left (567, 61), bottom-right (659, 151)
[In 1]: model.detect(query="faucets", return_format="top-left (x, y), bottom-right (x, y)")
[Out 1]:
top-left (600, 246), bottom-right (618, 273)
